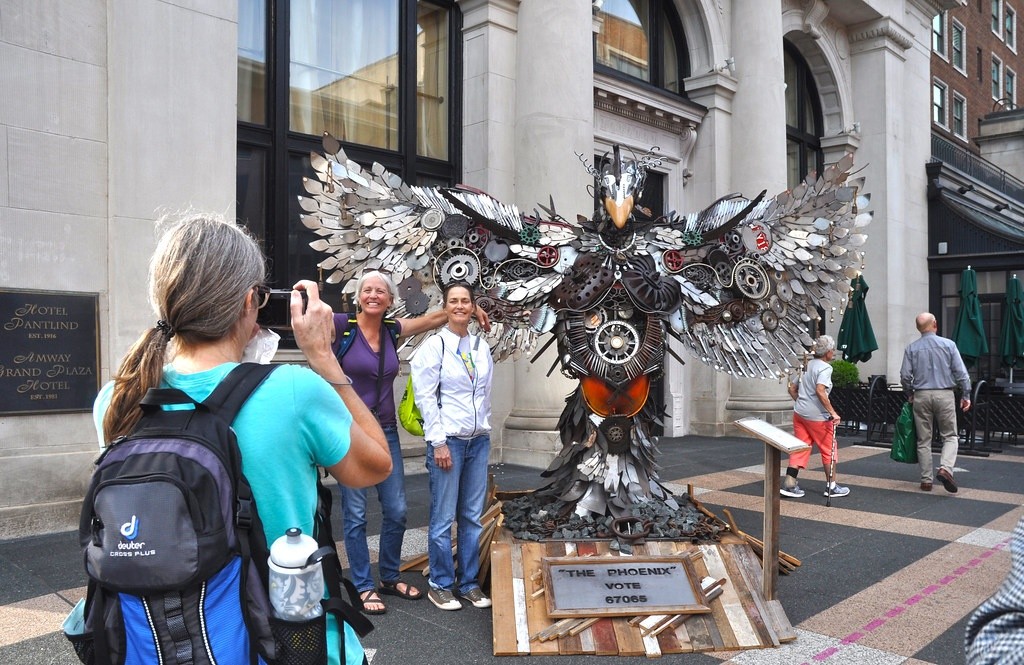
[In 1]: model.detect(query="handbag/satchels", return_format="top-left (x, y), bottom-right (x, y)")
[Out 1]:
top-left (398, 334), bottom-right (445, 437)
top-left (890, 402), bottom-right (919, 464)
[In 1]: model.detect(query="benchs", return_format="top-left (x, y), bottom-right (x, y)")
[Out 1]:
top-left (996, 382), bottom-right (1024, 448)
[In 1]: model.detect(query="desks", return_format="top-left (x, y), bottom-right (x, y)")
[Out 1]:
top-left (860, 386), bottom-right (903, 390)
top-left (860, 382), bottom-right (899, 385)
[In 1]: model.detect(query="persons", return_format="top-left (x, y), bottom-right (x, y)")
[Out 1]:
top-left (61, 216), bottom-right (393, 665)
top-left (327, 270), bottom-right (493, 614)
top-left (900, 312), bottom-right (972, 493)
top-left (778, 335), bottom-right (851, 498)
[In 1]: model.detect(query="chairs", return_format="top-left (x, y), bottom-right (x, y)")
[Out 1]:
top-left (869, 374), bottom-right (887, 387)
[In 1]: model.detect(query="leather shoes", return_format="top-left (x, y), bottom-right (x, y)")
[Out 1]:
top-left (936, 469), bottom-right (958, 493)
top-left (920, 480), bottom-right (933, 491)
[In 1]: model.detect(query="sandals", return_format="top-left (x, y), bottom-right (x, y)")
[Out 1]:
top-left (358, 589), bottom-right (387, 615)
top-left (377, 578), bottom-right (421, 600)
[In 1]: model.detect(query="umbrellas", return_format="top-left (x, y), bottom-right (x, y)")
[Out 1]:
top-left (838, 273), bottom-right (879, 364)
top-left (949, 265), bottom-right (1024, 397)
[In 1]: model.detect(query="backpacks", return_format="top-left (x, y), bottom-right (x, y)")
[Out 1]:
top-left (61, 362), bottom-right (375, 665)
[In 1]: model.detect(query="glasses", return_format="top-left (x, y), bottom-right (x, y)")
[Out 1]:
top-left (249, 285), bottom-right (268, 310)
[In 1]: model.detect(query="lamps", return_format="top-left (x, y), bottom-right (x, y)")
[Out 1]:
top-left (993, 203), bottom-right (1010, 212)
top-left (592, 0), bottom-right (604, 12)
top-left (719, 57), bottom-right (737, 73)
top-left (957, 184), bottom-right (974, 195)
top-left (847, 122), bottom-right (861, 134)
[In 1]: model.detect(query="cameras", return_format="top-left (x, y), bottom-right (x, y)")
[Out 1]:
top-left (258, 289), bottom-right (307, 330)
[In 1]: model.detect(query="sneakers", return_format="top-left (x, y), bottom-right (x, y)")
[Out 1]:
top-left (458, 586), bottom-right (491, 608)
top-left (428, 585), bottom-right (462, 610)
top-left (780, 481), bottom-right (806, 498)
top-left (823, 485), bottom-right (850, 498)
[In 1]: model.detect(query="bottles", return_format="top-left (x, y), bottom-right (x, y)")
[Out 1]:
top-left (267, 528), bottom-right (336, 621)
